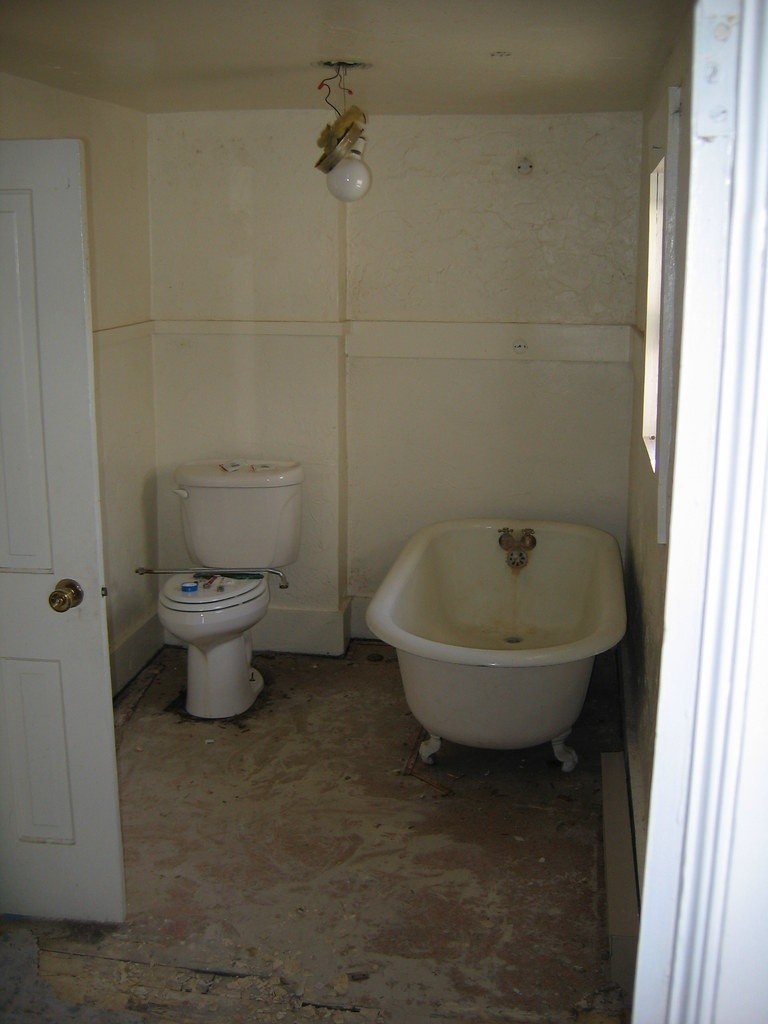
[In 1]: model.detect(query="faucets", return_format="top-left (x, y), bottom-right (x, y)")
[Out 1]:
top-left (499, 531), bottom-right (536, 564)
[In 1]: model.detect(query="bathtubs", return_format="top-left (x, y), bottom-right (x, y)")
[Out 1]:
top-left (362, 518), bottom-right (628, 751)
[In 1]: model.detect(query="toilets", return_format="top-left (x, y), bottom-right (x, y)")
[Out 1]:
top-left (157, 461), bottom-right (304, 720)
